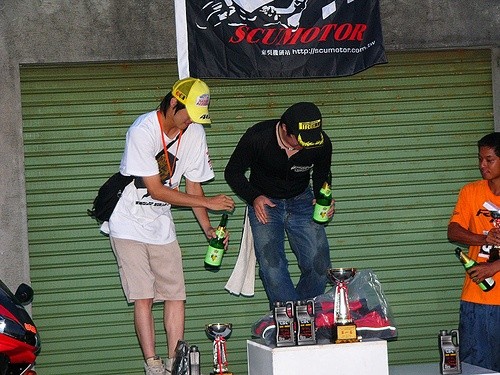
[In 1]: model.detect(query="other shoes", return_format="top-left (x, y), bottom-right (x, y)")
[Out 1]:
top-left (163, 358), bottom-right (174, 374)
top-left (144, 361), bottom-right (164, 375)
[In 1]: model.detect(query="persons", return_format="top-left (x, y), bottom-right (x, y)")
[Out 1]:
top-left (223, 102), bottom-right (335, 313)
top-left (446, 132), bottom-right (500, 373)
top-left (108, 77), bottom-right (236, 375)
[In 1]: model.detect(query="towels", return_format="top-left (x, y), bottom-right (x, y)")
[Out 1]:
top-left (223, 207), bottom-right (258, 298)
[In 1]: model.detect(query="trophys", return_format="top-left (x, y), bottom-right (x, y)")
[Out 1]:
top-left (326, 268), bottom-right (359, 344)
top-left (205, 323), bottom-right (233, 375)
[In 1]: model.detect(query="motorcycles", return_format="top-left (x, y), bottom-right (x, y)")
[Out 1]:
top-left (0, 279), bottom-right (42, 375)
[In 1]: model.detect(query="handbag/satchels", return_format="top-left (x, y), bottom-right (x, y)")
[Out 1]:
top-left (93, 172), bottom-right (134, 222)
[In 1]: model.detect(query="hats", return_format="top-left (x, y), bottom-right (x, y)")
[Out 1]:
top-left (171, 77), bottom-right (211, 124)
top-left (283, 101), bottom-right (324, 148)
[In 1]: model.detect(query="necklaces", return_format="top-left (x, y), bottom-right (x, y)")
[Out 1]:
top-left (278, 125), bottom-right (295, 151)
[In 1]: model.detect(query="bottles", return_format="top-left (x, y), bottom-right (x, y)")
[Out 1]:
top-left (313, 174), bottom-right (335, 225)
top-left (492, 215), bottom-right (500, 258)
top-left (296, 299), bottom-right (317, 346)
top-left (275, 301), bottom-right (296, 347)
top-left (439, 329), bottom-right (462, 375)
top-left (203, 213), bottom-right (230, 272)
top-left (454, 246), bottom-right (495, 293)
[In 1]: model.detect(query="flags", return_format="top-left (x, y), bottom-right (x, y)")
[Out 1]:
top-left (174, 0), bottom-right (388, 80)
